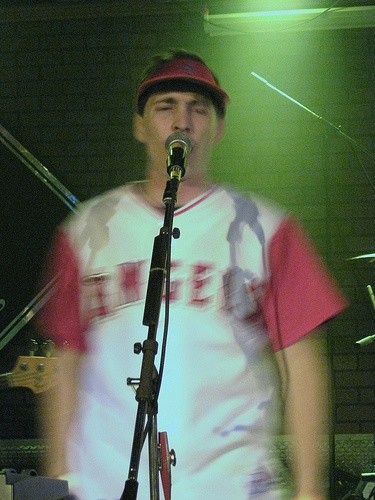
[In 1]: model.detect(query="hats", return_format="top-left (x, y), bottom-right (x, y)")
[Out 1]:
top-left (136, 50), bottom-right (230, 116)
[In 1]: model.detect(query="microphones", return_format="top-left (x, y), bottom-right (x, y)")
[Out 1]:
top-left (165, 132), bottom-right (193, 191)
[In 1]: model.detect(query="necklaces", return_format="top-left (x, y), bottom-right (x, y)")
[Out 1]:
top-left (135, 180), bottom-right (169, 210)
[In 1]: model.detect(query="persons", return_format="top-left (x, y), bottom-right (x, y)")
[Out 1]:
top-left (31, 47), bottom-right (347, 500)
